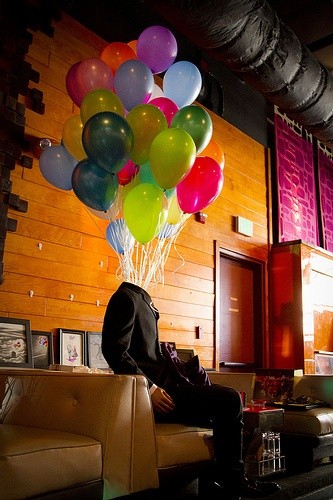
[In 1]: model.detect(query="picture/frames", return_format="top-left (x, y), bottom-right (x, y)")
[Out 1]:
top-left (86, 331), bottom-right (112, 370)
top-left (0, 316), bottom-right (34, 369)
top-left (31, 330), bottom-right (54, 370)
top-left (56, 328), bottom-right (86, 366)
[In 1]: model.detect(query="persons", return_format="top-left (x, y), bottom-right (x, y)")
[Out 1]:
top-left (101, 280), bottom-right (282, 499)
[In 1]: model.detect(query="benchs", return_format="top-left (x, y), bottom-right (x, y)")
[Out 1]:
top-left (0, 374), bottom-right (333, 500)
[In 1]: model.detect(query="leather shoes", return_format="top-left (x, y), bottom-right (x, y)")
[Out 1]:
top-left (198, 474), bottom-right (279, 500)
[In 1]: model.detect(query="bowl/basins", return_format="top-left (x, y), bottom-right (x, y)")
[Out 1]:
top-left (251, 399), bottom-right (266, 407)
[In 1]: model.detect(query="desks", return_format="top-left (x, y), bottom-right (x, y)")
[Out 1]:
top-left (241, 404), bottom-right (284, 457)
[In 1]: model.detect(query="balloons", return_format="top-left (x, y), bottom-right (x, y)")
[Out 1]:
top-left (39, 25), bottom-right (224, 255)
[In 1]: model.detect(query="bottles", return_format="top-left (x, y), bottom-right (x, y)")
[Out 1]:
top-left (259, 456), bottom-right (285, 475)
top-left (262, 432), bottom-right (281, 459)
